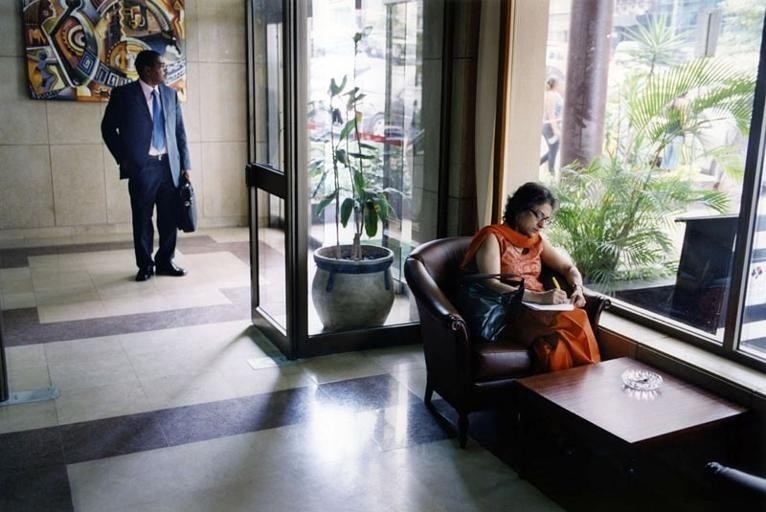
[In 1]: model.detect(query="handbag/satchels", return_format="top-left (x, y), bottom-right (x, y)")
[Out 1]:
top-left (176, 174), bottom-right (197, 234)
top-left (453, 273), bottom-right (524, 346)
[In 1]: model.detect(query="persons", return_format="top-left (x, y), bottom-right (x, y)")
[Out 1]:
top-left (540, 74), bottom-right (567, 174)
top-left (101, 50), bottom-right (193, 282)
top-left (454, 181), bottom-right (600, 377)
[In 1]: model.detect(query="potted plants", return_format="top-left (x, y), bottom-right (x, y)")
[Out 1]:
top-left (307, 27), bottom-right (407, 332)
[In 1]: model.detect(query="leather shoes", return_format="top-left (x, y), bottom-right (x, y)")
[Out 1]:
top-left (135, 265), bottom-right (154, 281)
top-left (155, 260), bottom-right (186, 276)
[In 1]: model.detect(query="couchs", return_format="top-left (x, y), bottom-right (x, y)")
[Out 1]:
top-left (402, 234), bottom-right (611, 448)
top-left (688, 448), bottom-right (765, 512)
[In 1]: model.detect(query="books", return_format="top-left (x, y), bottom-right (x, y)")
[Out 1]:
top-left (516, 299), bottom-right (578, 313)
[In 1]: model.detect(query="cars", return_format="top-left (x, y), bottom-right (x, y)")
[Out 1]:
top-left (361, 25), bottom-right (422, 64)
top-left (324, 61), bottom-right (420, 145)
top-left (632, 113), bottom-right (744, 198)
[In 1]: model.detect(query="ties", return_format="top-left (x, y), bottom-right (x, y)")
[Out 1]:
top-left (151, 90), bottom-right (166, 149)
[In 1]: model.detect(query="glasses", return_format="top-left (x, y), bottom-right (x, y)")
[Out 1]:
top-left (528, 209), bottom-right (551, 222)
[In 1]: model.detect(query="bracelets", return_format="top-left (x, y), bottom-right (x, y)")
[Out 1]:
top-left (569, 283), bottom-right (582, 290)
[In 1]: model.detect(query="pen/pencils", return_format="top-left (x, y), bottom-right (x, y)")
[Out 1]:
top-left (550, 272), bottom-right (562, 290)
